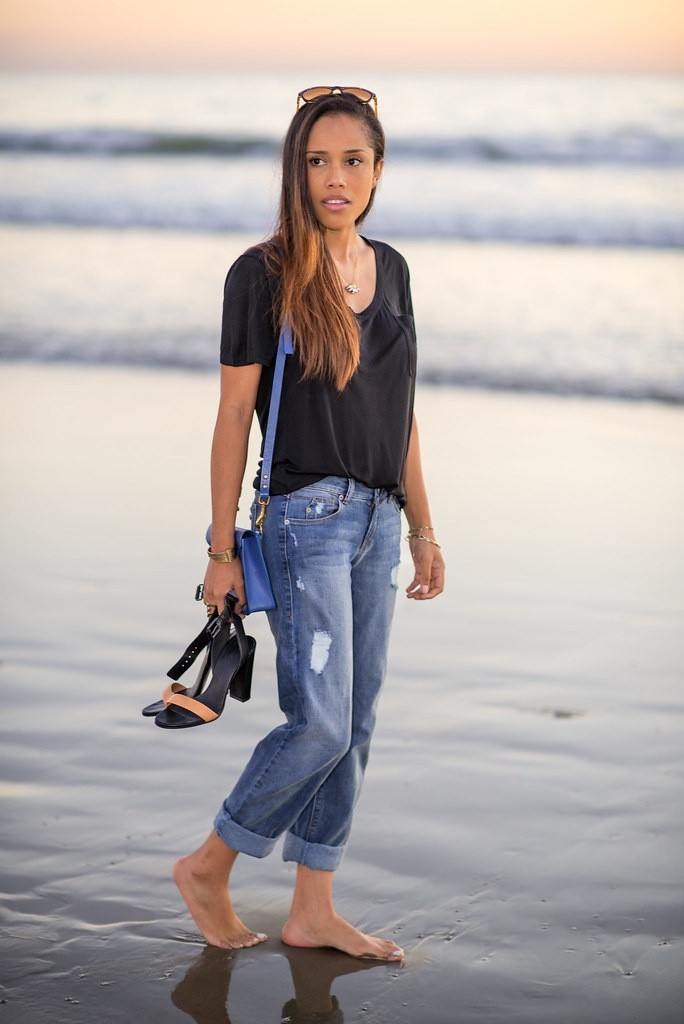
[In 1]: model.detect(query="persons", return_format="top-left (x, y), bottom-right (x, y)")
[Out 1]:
top-left (170, 946), bottom-right (405, 1023)
top-left (170, 88), bottom-right (446, 962)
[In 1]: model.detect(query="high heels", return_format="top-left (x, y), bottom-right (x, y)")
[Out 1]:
top-left (142, 607), bottom-right (256, 729)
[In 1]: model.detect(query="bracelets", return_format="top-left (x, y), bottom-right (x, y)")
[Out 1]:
top-left (405, 527), bottom-right (441, 546)
top-left (207, 547), bottom-right (239, 562)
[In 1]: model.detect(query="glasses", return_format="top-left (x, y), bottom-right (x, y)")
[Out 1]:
top-left (296, 88), bottom-right (379, 120)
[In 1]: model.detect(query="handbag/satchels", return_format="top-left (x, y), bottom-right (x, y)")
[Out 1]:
top-left (206, 521), bottom-right (276, 615)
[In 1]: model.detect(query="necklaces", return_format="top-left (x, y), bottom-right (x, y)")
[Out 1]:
top-left (326, 257), bottom-right (365, 293)
